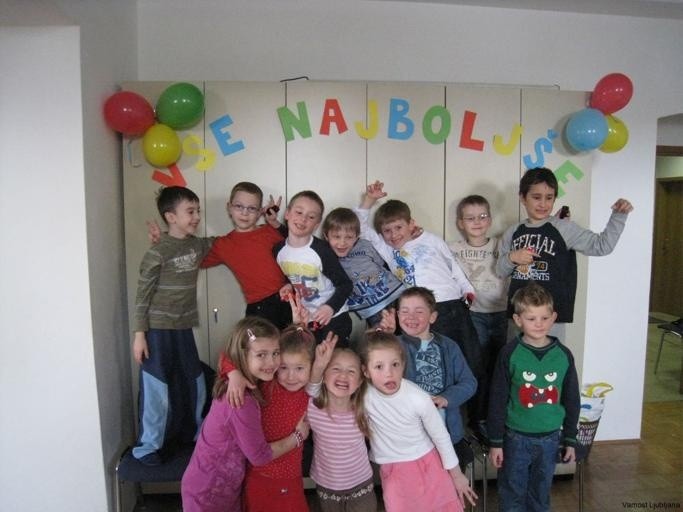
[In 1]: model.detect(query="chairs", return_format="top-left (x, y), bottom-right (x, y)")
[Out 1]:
top-left (113, 359), bottom-right (218, 512)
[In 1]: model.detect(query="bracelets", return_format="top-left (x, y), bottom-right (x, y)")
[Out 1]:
top-left (289, 429), bottom-right (304, 448)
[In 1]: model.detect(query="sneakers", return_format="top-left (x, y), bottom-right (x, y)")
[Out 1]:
top-left (467, 420), bottom-right (489, 445)
top-left (129, 448), bottom-right (164, 466)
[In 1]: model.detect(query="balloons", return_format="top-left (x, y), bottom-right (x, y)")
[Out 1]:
top-left (591, 73), bottom-right (633, 114)
top-left (104, 90), bottom-right (155, 135)
top-left (564, 107), bottom-right (608, 152)
top-left (141, 122), bottom-right (182, 167)
top-left (599, 114), bottom-right (629, 154)
top-left (154, 81), bottom-right (205, 128)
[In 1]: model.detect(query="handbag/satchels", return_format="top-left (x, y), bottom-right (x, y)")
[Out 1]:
top-left (580, 382), bottom-right (614, 446)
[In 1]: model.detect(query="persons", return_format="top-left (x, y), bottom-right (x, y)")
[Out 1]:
top-left (495, 167), bottom-right (634, 346)
top-left (487, 282), bottom-right (581, 512)
top-left (379, 287), bottom-right (479, 475)
top-left (351, 331), bottom-right (479, 512)
top-left (264, 208), bottom-right (407, 337)
top-left (302, 331), bottom-right (379, 512)
top-left (351, 180), bottom-right (491, 454)
top-left (272, 189), bottom-right (353, 349)
top-left (241, 321), bottom-right (316, 512)
top-left (180, 316), bottom-right (310, 511)
top-left (440, 195), bottom-right (571, 414)
top-left (143, 181), bottom-right (295, 332)
top-left (132, 187), bottom-right (220, 468)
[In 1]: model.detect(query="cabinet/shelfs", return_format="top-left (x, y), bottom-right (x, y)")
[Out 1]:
top-left (121, 79), bottom-right (287, 444)
top-left (445, 82), bottom-right (595, 427)
top-left (286, 80), bottom-right (445, 350)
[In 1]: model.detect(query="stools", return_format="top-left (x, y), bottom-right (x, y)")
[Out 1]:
top-left (654, 317), bottom-right (683, 395)
top-left (468, 428), bottom-right (584, 512)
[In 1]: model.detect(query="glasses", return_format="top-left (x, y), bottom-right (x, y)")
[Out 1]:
top-left (232, 202), bottom-right (259, 213)
top-left (461, 213), bottom-right (489, 222)
